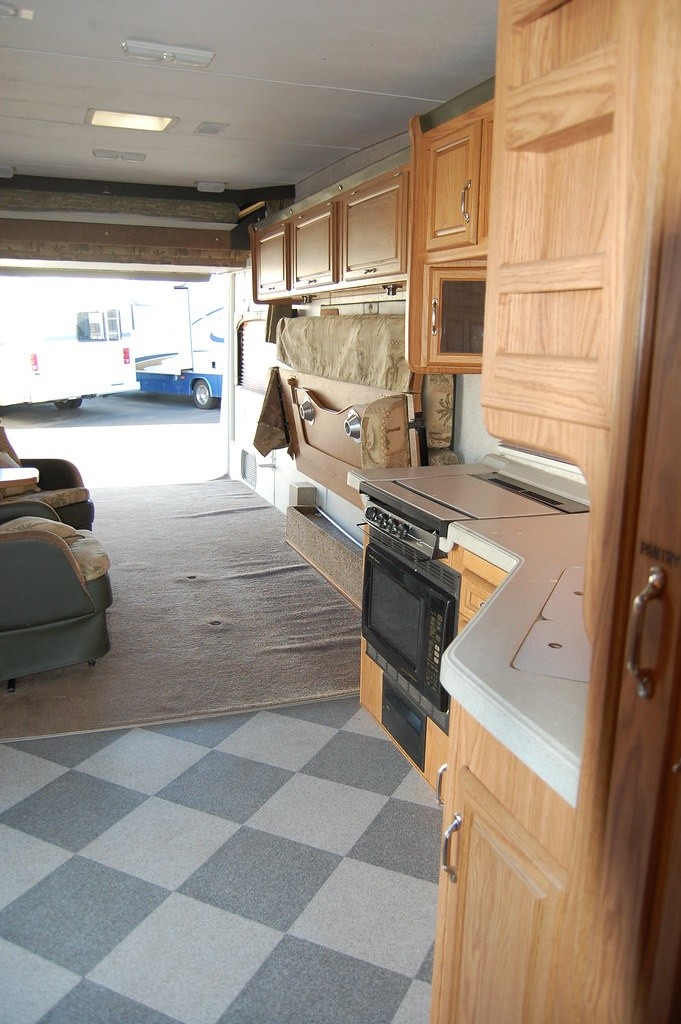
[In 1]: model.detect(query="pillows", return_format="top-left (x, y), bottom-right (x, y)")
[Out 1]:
top-left (0, 450), bottom-right (42, 497)
top-left (0, 515), bottom-right (85, 546)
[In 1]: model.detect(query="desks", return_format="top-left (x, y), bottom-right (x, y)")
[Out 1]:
top-left (0, 467), bottom-right (40, 489)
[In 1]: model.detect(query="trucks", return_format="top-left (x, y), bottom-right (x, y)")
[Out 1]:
top-left (135, 277), bottom-right (226, 409)
top-left (0, 259), bottom-right (140, 409)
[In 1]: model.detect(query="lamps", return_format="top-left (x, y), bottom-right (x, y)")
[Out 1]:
top-left (192, 179), bottom-right (230, 193)
top-left (123, 40), bottom-right (215, 69)
top-left (93, 150), bottom-right (147, 163)
top-left (0, 164), bottom-right (16, 178)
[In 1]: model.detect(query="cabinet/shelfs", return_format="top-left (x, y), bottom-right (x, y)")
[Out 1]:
top-left (251, 0), bottom-right (681, 1024)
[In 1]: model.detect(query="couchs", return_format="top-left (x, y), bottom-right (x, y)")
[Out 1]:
top-left (0, 424), bottom-right (113, 691)
